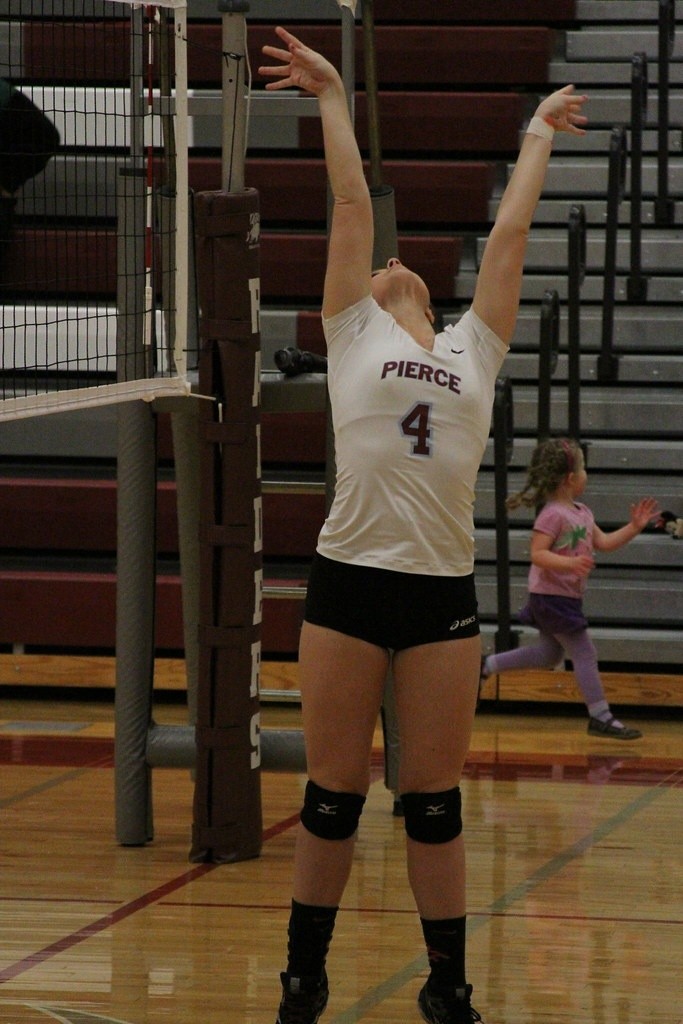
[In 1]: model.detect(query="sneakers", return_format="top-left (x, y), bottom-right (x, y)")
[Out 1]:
top-left (417, 973), bottom-right (482, 1024)
top-left (275, 960), bottom-right (328, 1024)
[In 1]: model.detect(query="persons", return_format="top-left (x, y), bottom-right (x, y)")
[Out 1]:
top-left (477, 437), bottom-right (662, 739)
top-left (258, 26), bottom-right (589, 1024)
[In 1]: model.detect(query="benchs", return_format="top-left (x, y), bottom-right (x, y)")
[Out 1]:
top-left (0, 0), bottom-right (575, 653)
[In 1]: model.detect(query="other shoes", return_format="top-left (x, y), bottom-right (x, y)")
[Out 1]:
top-left (585, 715), bottom-right (643, 741)
top-left (477, 653), bottom-right (488, 698)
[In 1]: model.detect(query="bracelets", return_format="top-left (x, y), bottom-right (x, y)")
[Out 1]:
top-left (525, 117), bottom-right (555, 141)
